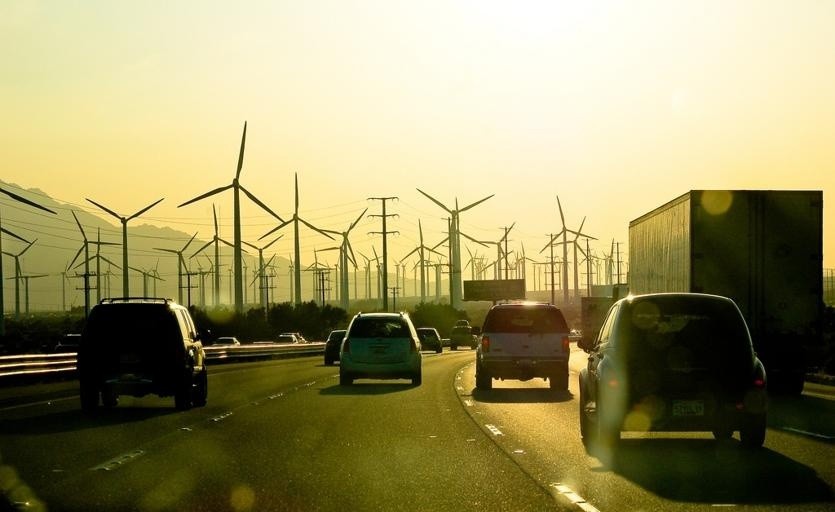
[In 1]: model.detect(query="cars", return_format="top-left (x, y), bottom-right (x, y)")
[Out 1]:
top-left (323, 330), bottom-right (346, 365)
top-left (415, 327), bottom-right (443, 354)
top-left (212, 336), bottom-right (240, 347)
top-left (54, 332), bottom-right (82, 353)
top-left (577, 292), bottom-right (767, 452)
top-left (276, 332), bottom-right (306, 345)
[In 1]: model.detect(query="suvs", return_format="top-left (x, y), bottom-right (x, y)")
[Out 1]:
top-left (474, 301), bottom-right (571, 393)
top-left (338, 309), bottom-right (423, 387)
top-left (74, 295), bottom-right (209, 412)
top-left (450, 319), bottom-right (479, 351)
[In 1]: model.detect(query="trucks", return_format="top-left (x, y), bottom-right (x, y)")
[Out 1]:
top-left (611, 190), bottom-right (825, 395)
